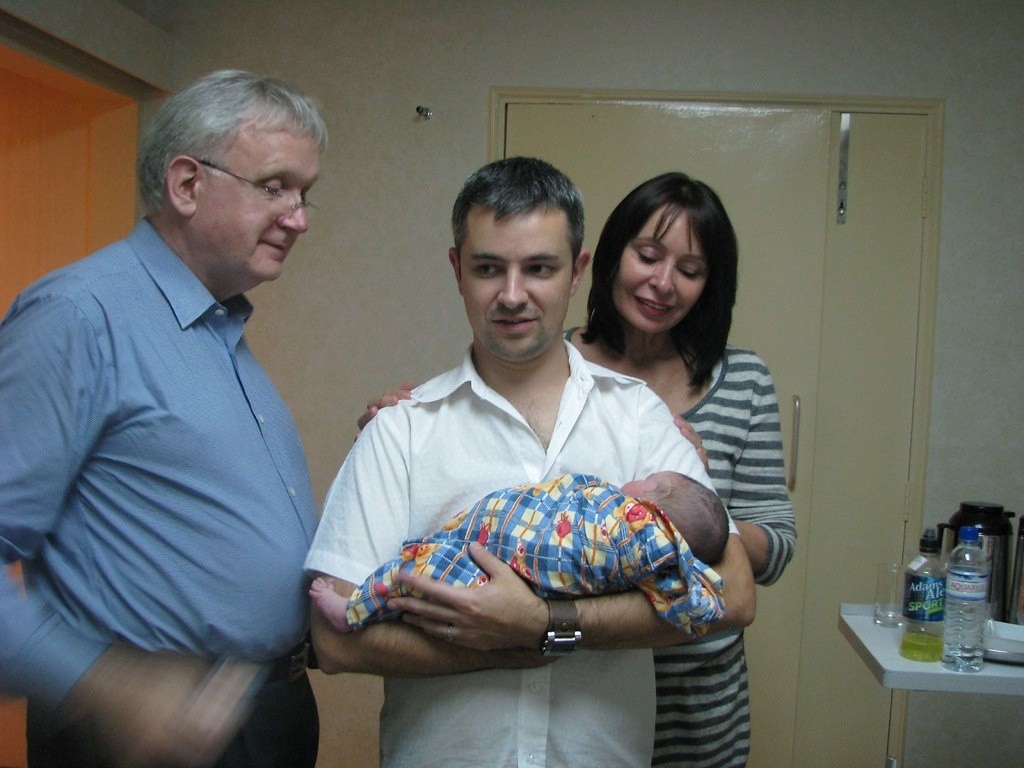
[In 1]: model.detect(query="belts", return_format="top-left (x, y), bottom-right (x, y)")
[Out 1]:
top-left (260, 640), bottom-right (311, 701)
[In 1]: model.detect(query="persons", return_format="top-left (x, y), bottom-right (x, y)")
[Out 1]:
top-left (0, 65), bottom-right (324, 768)
top-left (308, 470), bottom-right (728, 636)
top-left (353, 170), bottom-right (796, 768)
top-left (302, 153), bottom-right (754, 768)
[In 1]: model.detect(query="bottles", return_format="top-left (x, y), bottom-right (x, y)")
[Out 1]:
top-left (943, 525), bottom-right (989, 672)
top-left (899, 527), bottom-right (946, 662)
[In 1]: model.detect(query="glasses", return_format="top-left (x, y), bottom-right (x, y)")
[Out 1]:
top-left (199, 159), bottom-right (319, 226)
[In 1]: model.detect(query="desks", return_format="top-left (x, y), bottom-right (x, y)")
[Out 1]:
top-left (835, 602), bottom-right (1024, 696)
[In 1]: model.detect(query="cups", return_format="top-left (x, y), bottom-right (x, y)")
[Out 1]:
top-left (873, 561), bottom-right (906, 628)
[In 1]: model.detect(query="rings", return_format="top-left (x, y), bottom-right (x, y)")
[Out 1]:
top-left (447, 624), bottom-right (454, 641)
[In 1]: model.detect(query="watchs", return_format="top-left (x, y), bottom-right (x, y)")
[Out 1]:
top-left (539, 596), bottom-right (582, 658)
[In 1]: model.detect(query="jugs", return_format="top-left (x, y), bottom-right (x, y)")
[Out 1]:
top-left (935, 500), bottom-right (1024, 629)
